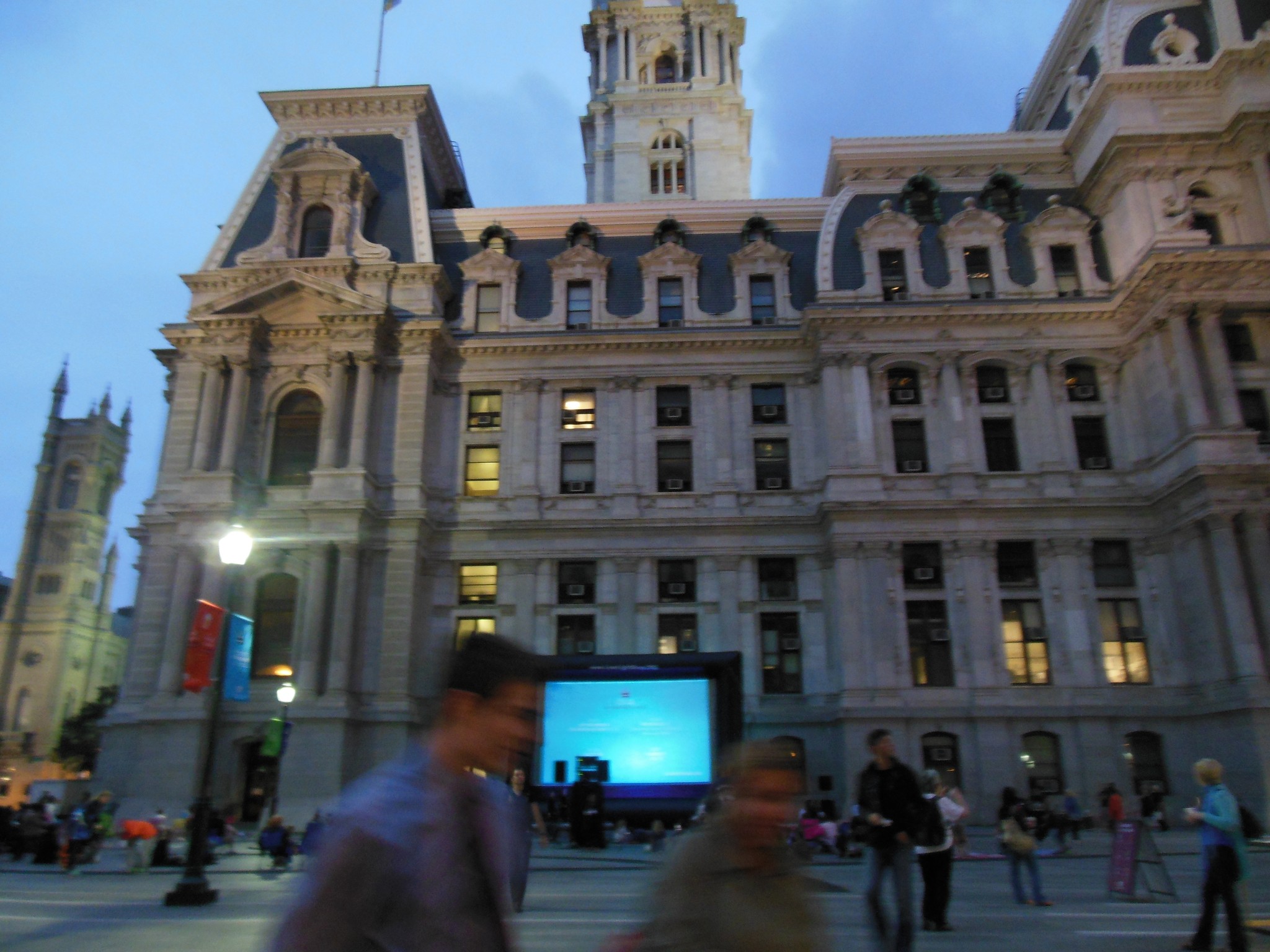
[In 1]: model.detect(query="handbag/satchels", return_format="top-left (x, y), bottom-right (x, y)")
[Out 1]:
top-left (1001, 821), bottom-right (1036, 852)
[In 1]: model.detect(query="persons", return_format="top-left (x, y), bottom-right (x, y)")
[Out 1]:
top-left (2, 787), bottom-right (321, 878)
top-left (606, 736), bottom-right (842, 952)
top-left (501, 766), bottom-right (545, 912)
top-left (850, 728), bottom-right (1256, 952)
top-left (261, 632), bottom-right (545, 952)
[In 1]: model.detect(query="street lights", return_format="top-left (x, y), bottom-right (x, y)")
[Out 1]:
top-left (163, 524), bottom-right (255, 905)
top-left (270, 682), bottom-right (295, 815)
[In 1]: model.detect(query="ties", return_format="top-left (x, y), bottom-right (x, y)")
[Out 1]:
top-left (456, 791), bottom-right (511, 952)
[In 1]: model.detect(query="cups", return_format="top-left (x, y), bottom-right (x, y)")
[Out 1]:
top-left (1185, 807), bottom-right (1196, 822)
top-left (1026, 817), bottom-right (1035, 827)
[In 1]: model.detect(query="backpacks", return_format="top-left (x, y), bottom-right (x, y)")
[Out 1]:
top-left (913, 797), bottom-right (946, 847)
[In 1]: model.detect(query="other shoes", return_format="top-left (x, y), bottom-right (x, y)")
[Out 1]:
top-left (1034, 898), bottom-right (1053, 906)
top-left (922, 922), bottom-right (955, 932)
